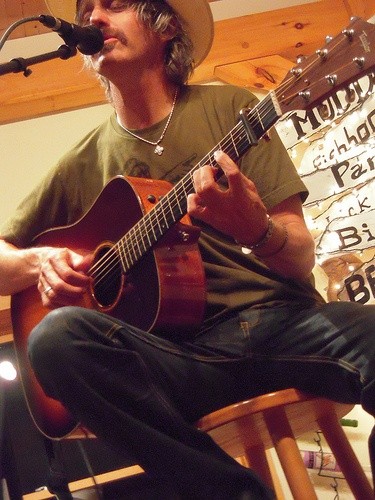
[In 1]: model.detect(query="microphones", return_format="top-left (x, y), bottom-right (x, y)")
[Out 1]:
top-left (39, 13), bottom-right (104, 56)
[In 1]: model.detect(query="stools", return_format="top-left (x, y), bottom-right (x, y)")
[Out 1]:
top-left (194, 386), bottom-right (375, 500)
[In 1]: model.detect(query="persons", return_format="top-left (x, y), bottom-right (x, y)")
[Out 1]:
top-left (0, 0), bottom-right (375, 500)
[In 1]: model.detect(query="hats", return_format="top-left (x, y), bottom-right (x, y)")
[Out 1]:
top-left (44, 0), bottom-right (216, 69)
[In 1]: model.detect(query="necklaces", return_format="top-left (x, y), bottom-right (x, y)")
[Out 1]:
top-left (113, 81), bottom-right (179, 156)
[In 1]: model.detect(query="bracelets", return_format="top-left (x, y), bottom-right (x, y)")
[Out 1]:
top-left (252, 219), bottom-right (289, 259)
top-left (234, 212), bottom-right (274, 251)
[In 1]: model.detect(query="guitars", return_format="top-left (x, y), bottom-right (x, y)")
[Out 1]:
top-left (8, 15), bottom-right (375, 441)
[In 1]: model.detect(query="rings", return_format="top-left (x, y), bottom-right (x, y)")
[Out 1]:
top-left (43, 286), bottom-right (52, 296)
